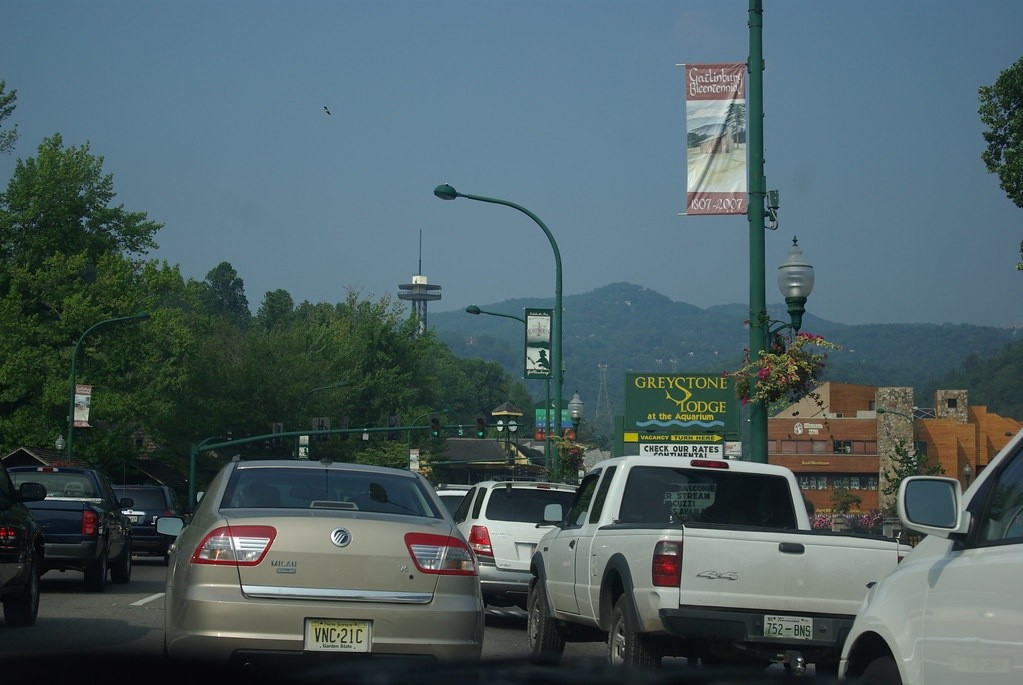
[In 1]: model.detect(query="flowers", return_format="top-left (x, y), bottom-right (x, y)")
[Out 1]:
top-left (723, 316), bottom-right (842, 448)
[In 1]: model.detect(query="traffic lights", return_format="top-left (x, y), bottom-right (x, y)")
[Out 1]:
top-left (477, 418), bottom-right (484, 439)
top-left (432, 418), bottom-right (440, 437)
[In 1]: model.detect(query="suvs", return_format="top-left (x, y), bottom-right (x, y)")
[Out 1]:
top-left (453, 478), bottom-right (579, 613)
top-left (7, 465), bottom-right (135, 594)
top-left (435, 482), bottom-right (473, 520)
top-left (112, 484), bottom-right (194, 563)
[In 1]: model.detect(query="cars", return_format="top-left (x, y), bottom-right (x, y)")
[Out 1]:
top-left (155, 461), bottom-right (484, 685)
top-left (836, 423), bottom-right (1023, 685)
top-left (0, 459), bottom-right (48, 628)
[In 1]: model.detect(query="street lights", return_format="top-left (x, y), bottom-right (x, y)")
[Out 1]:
top-left (294, 381), bottom-right (349, 458)
top-left (466, 305), bottom-right (551, 474)
top-left (407, 409), bottom-right (449, 471)
top-left (434, 182), bottom-right (563, 484)
top-left (748, 235), bottom-right (815, 464)
top-left (874, 408), bottom-right (920, 476)
top-left (66, 312), bottom-right (152, 466)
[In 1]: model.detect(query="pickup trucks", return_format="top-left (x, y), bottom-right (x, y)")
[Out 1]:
top-left (527, 455), bottom-right (914, 685)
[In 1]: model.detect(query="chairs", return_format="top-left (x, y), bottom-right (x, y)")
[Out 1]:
top-left (240, 483), bottom-right (279, 508)
top-left (63, 482), bottom-right (84, 496)
